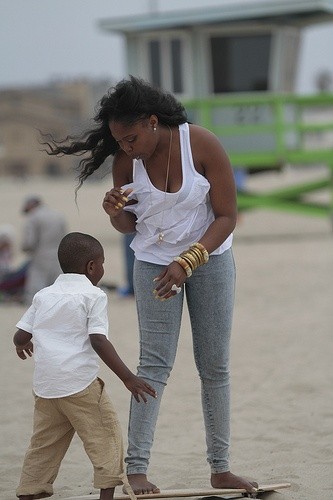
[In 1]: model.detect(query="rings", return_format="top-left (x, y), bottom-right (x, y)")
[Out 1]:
top-left (171, 284), bottom-right (182, 294)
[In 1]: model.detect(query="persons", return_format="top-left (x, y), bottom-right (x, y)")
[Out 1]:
top-left (0, 195), bottom-right (69, 304)
top-left (48, 78), bottom-right (260, 496)
top-left (13, 231), bottom-right (158, 500)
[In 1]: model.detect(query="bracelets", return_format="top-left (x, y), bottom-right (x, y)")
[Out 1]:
top-left (175, 243), bottom-right (209, 278)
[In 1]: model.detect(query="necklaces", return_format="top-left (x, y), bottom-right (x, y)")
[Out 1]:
top-left (143, 126), bottom-right (173, 244)
